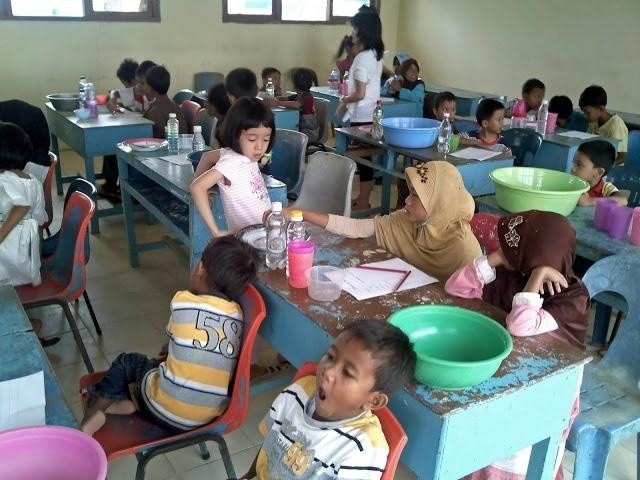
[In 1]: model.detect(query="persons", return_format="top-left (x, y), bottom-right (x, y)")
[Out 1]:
top-left (264, 161), bottom-right (483, 278)
top-left (504, 78), bottom-right (630, 167)
top-left (571, 140), bottom-right (628, 208)
top-left (0, 121), bottom-right (50, 336)
top-left (100, 59), bottom-right (319, 206)
top-left (255, 319), bottom-right (416, 480)
top-left (444, 209), bottom-right (591, 479)
top-left (373, 52), bottom-right (426, 185)
top-left (336, 36), bottom-right (357, 78)
top-left (190, 96), bottom-right (276, 243)
top-left (335, 10), bottom-right (384, 210)
top-left (433, 91), bottom-right (509, 155)
top-left (79, 235), bottom-right (260, 438)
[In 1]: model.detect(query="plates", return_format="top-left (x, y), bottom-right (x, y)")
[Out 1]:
top-left (123, 138), bottom-right (169, 151)
top-left (359, 125), bottom-right (383, 137)
top-left (234, 224), bottom-right (311, 252)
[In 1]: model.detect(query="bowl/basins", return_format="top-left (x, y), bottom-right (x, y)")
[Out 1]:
top-left (304, 267), bottom-right (347, 302)
top-left (177, 134), bottom-right (193, 151)
top-left (46, 94), bottom-right (80, 112)
top-left (73, 109), bottom-right (91, 119)
top-left (0, 426), bottom-right (109, 480)
top-left (186, 150), bottom-right (215, 172)
top-left (381, 117), bottom-right (441, 149)
top-left (489, 165), bottom-right (591, 219)
top-left (388, 305), bottom-right (514, 392)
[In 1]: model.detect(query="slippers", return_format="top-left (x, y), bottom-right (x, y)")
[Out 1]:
top-left (37, 335), bottom-right (61, 349)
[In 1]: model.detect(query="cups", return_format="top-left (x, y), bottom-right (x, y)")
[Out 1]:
top-left (594, 197), bottom-right (640, 246)
top-left (509, 99), bottom-right (527, 129)
top-left (546, 112), bottom-right (558, 134)
top-left (96, 95), bottom-right (106, 105)
top-left (528, 115), bottom-right (536, 123)
top-left (286, 240), bottom-right (315, 289)
top-left (450, 136), bottom-right (459, 152)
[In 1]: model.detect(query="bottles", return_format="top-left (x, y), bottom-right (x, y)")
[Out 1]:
top-left (191, 125), bottom-right (206, 153)
top-left (167, 113), bottom-right (179, 152)
top-left (328, 65), bottom-right (349, 96)
top-left (265, 201), bottom-right (286, 271)
top-left (285, 210), bottom-right (305, 277)
top-left (535, 100), bottom-right (549, 135)
top-left (372, 100), bottom-right (384, 140)
top-left (78, 76), bottom-right (97, 118)
top-left (479, 96), bottom-right (486, 103)
top-left (437, 113), bottom-right (451, 153)
top-left (266, 78), bottom-right (275, 98)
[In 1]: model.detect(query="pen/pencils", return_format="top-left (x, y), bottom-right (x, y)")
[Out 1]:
top-left (396, 270), bottom-right (412, 292)
top-left (355, 264), bottom-right (408, 273)
